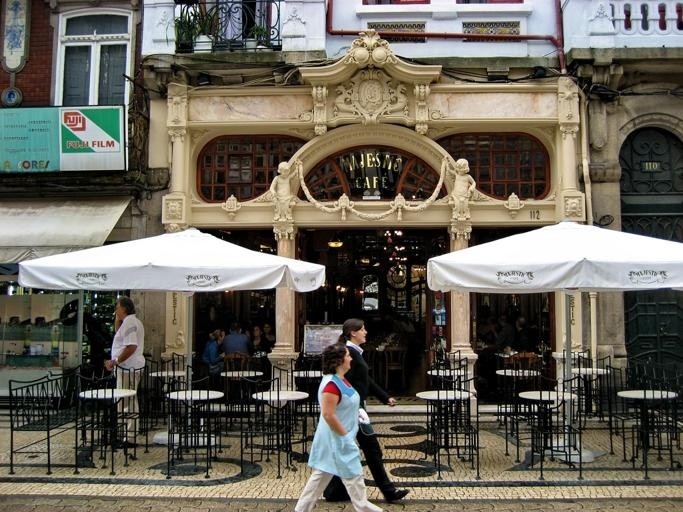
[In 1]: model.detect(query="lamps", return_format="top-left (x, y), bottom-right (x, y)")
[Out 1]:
top-left (326, 230), bottom-right (343, 248)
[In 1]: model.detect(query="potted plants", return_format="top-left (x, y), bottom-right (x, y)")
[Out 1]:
top-left (248, 23), bottom-right (274, 52)
top-left (163, 10), bottom-right (222, 54)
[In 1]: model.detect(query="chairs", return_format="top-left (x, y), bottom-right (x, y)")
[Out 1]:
top-left (415, 338), bottom-right (683, 481)
top-left (9, 338), bottom-right (323, 480)
top-left (360, 339), bottom-right (406, 389)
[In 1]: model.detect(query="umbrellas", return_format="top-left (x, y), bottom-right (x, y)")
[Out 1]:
top-left (18, 227), bottom-right (326, 438)
top-left (426, 222), bottom-right (683, 447)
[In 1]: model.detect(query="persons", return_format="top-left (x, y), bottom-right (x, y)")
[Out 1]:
top-left (295, 343), bottom-right (383, 512)
top-left (323, 318), bottom-right (410, 503)
top-left (200, 322), bottom-right (276, 378)
top-left (477, 316), bottom-right (536, 353)
top-left (445, 158), bottom-right (476, 219)
top-left (270, 162), bottom-right (299, 220)
top-left (104, 296), bottom-right (145, 448)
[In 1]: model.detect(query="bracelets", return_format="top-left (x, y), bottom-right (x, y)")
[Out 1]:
top-left (116, 357), bottom-right (120, 365)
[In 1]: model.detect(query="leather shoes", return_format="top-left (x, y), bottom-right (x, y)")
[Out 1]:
top-left (386, 489), bottom-right (410, 503)
